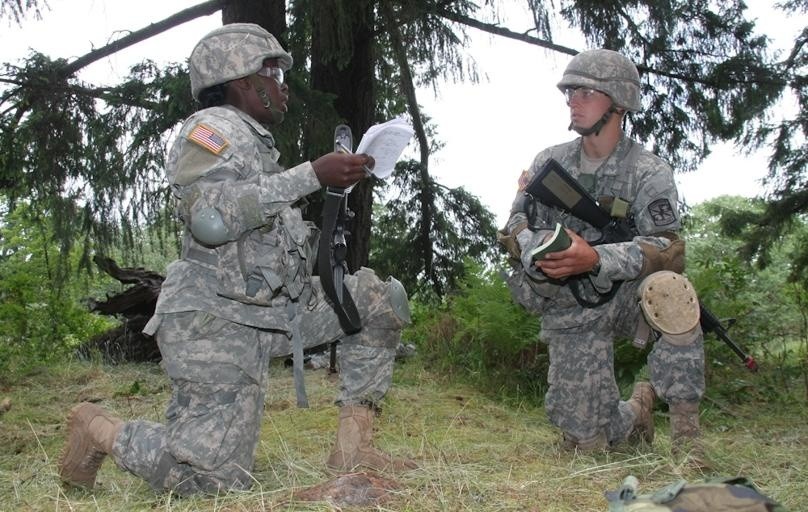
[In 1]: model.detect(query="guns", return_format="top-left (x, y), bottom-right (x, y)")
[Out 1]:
top-left (524, 159), bottom-right (761, 375)
top-left (331, 125), bottom-right (353, 372)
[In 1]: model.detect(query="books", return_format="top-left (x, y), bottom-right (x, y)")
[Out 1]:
top-left (355, 118), bottom-right (416, 180)
top-left (532, 222), bottom-right (582, 281)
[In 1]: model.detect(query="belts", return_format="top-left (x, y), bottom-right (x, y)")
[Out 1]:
top-left (316, 184), bottom-right (363, 335)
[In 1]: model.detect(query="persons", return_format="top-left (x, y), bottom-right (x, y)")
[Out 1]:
top-left (58, 22), bottom-right (419, 501)
top-left (495, 48), bottom-right (712, 470)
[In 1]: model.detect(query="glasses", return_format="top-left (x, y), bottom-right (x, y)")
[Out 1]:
top-left (562, 88), bottom-right (598, 100)
top-left (258, 67), bottom-right (286, 85)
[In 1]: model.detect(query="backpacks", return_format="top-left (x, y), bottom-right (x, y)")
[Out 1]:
top-left (605, 476), bottom-right (782, 512)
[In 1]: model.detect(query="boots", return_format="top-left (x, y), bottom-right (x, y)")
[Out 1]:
top-left (626, 381), bottom-right (658, 446)
top-left (669, 402), bottom-right (719, 479)
top-left (59, 401), bottom-right (120, 493)
top-left (328, 406), bottom-right (418, 471)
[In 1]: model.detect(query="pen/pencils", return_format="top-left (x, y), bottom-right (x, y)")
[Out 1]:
top-left (335, 140), bottom-right (381, 184)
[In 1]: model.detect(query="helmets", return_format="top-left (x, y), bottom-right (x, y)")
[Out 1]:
top-left (190, 23), bottom-right (292, 103)
top-left (557, 48), bottom-right (641, 111)
